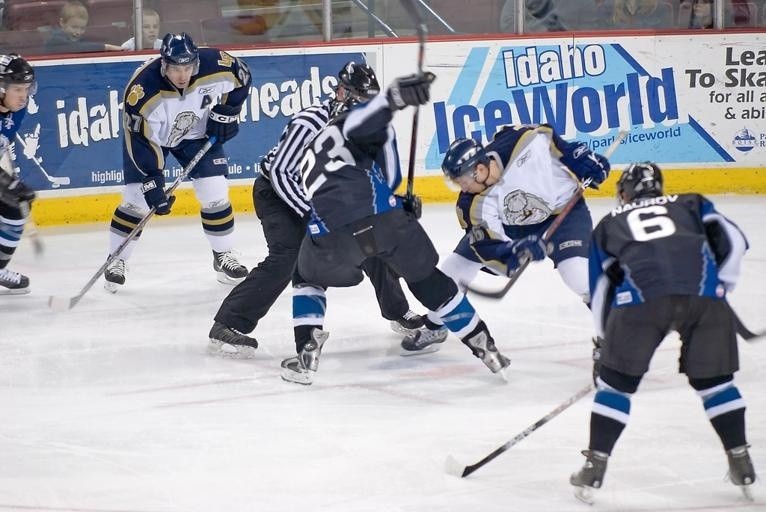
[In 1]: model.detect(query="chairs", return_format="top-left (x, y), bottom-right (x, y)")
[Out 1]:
top-left (0, 1), bottom-right (272, 62)
top-left (425, 0), bottom-right (766, 42)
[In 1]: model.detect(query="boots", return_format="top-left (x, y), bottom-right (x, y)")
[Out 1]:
top-left (280, 326), bottom-right (330, 376)
top-left (400, 321), bottom-right (449, 352)
top-left (568, 450), bottom-right (610, 490)
top-left (207, 320), bottom-right (259, 349)
top-left (726, 444), bottom-right (757, 487)
top-left (103, 253), bottom-right (126, 286)
top-left (211, 249), bottom-right (249, 279)
top-left (394, 309), bottom-right (426, 329)
top-left (0, 268), bottom-right (31, 290)
top-left (461, 319), bottom-right (506, 374)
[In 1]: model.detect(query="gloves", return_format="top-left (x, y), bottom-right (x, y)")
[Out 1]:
top-left (494, 234), bottom-right (547, 271)
top-left (384, 71), bottom-right (437, 113)
top-left (591, 337), bottom-right (606, 388)
top-left (204, 103), bottom-right (242, 145)
top-left (139, 168), bottom-right (177, 216)
top-left (6, 177), bottom-right (37, 204)
top-left (401, 194), bottom-right (423, 220)
top-left (560, 141), bottom-right (612, 191)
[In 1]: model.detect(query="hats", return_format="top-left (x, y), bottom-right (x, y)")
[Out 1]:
top-left (0, 52), bottom-right (35, 85)
top-left (615, 160), bottom-right (664, 201)
top-left (336, 60), bottom-right (382, 99)
top-left (159, 31), bottom-right (199, 66)
top-left (439, 136), bottom-right (492, 176)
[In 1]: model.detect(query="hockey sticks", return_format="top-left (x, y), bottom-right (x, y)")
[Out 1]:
top-left (432, 385), bottom-right (595, 478)
top-left (49, 136), bottom-right (217, 310)
top-left (458, 130), bottom-right (627, 298)
top-left (14, 131), bottom-right (71, 185)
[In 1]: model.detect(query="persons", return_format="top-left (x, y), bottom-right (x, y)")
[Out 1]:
top-left (0, 51), bottom-right (36, 291)
top-left (499, 0), bottom-right (714, 42)
top-left (569, 157), bottom-right (766, 491)
top-left (104, 32), bottom-right (252, 285)
top-left (402, 123), bottom-right (611, 352)
top-left (43, 0), bottom-right (128, 53)
top-left (209, 60), bottom-right (425, 349)
top-left (122, 9), bottom-right (163, 50)
top-left (279, 72), bottom-right (511, 375)
